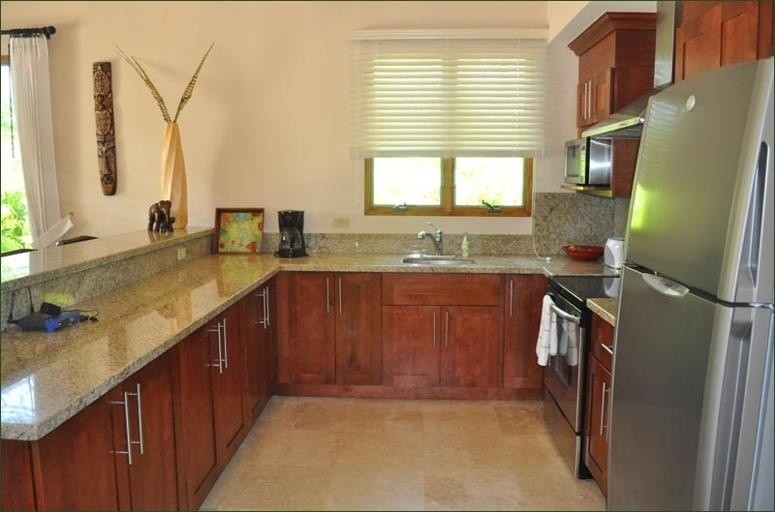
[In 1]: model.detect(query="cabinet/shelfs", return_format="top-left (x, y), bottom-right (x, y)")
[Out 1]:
top-left (581, 311), bottom-right (615, 497)
top-left (568, 12), bottom-right (657, 200)
top-left (499, 273), bottom-right (546, 402)
top-left (279, 272), bottom-right (504, 401)
top-left (671, 0), bottom-right (775, 87)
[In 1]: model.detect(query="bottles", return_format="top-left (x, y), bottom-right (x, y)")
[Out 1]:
top-left (461, 233), bottom-right (469, 258)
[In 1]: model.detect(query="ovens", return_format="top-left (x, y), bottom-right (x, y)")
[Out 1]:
top-left (539, 285), bottom-right (587, 436)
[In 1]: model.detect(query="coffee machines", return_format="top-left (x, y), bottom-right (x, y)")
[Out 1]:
top-left (277, 210), bottom-right (306, 257)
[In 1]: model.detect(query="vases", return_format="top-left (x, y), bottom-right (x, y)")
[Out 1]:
top-left (160, 122), bottom-right (188, 229)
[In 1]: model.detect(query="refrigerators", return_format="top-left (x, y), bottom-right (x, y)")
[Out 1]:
top-left (607, 57), bottom-right (775, 512)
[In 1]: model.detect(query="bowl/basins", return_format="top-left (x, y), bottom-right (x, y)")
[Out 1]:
top-left (561, 245), bottom-right (602, 261)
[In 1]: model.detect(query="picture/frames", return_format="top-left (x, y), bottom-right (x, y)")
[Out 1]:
top-left (213, 206), bottom-right (264, 255)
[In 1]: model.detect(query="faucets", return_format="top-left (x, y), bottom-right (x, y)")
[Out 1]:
top-left (417, 222), bottom-right (443, 255)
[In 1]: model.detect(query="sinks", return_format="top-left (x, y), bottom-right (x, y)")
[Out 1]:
top-left (401, 252), bottom-right (476, 267)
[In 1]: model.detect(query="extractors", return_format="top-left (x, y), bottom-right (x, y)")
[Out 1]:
top-left (581, 0), bottom-right (682, 140)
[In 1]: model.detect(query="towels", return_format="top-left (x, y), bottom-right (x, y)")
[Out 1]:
top-left (534, 295), bottom-right (559, 368)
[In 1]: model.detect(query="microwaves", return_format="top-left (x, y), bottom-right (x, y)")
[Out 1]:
top-left (564, 136), bottom-right (611, 186)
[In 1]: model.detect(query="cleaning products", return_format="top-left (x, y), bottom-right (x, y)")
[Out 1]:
top-left (460, 231), bottom-right (470, 258)
